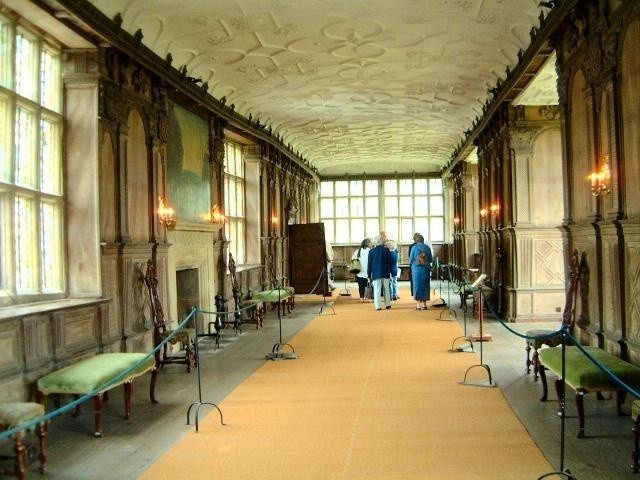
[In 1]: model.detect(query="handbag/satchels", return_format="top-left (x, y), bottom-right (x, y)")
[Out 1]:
top-left (416, 252), bottom-right (426, 267)
top-left (364, 281), bottom-right (373, 299)
top-left (349, 249), bottom-right (362, 274)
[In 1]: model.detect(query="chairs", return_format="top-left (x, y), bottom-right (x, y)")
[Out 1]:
top-left (268, 252), bottom-right (295, 310)
top-left (525, 254), bottom-right (581, 382)
top-left (0, 402), bottom-right (49, 480)
top-left (228, 252), bottom-right (264, 331)
top-left (472, 248), bottom-right (503, 318)
top-left (144, 258), bottom-right (199, 373)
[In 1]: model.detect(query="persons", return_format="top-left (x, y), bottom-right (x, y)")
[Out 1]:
top-left (350, 232), bottom-right (433, 310)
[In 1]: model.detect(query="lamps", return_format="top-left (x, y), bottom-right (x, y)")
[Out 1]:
top-left (590, 173), bottom-right (611, 198)
top-left (158, 195), bottom-right (174, 226)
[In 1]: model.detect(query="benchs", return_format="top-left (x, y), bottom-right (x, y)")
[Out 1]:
top-left (252, 289), bottom-right (292, 315)
top-left (461, 283), bottom-right (493, 316)
top-left (32, 352), bottom-right (160, 439)
top-left (535, 346), bottom-right (639, 439)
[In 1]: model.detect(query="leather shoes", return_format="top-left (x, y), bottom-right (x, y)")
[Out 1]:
top-left (376, 305), bottom-right (427, 311)
